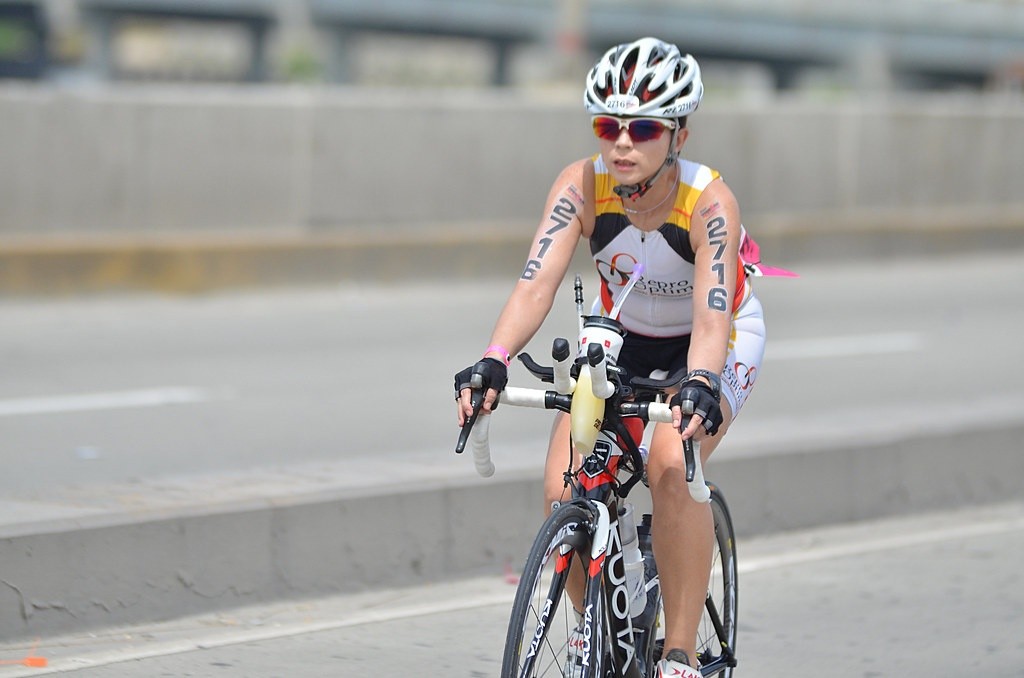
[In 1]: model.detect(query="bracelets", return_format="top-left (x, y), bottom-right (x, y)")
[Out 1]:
top-left (482, 343), bottom-right (510, 367)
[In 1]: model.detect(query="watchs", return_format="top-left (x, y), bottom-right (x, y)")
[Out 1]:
top-left (687, 367), bottom-right (722, 404)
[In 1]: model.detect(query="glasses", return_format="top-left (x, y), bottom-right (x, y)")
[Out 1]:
top-left (588, 115), bottom-right (680, 142)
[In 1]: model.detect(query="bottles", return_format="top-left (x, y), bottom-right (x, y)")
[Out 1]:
top-left (628, 513), bottom-right (662, 625)
top-left (615, 503), bottom-right (647, 618)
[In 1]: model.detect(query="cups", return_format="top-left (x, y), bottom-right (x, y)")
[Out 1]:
top-left (571, 313), bottom-right (628, 455)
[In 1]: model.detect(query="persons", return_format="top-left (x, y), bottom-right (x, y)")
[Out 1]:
top-left (451, 35), bottom-right (767, 677)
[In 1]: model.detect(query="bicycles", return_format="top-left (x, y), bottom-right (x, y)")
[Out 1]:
top-left (453, 274), bottom-right (738, 678)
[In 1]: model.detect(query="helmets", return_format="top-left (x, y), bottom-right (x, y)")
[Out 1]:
top-left (583, 36), bottom-right (705, 117)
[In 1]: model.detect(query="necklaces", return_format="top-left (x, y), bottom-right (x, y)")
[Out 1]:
top-left (622, 162), bottom-right (681, 215)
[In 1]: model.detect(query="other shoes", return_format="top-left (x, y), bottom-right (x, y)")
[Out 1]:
top-left (563, 606), bottom-right (592, 678)
top-left (656, 657), bottom-right (704, 678)
